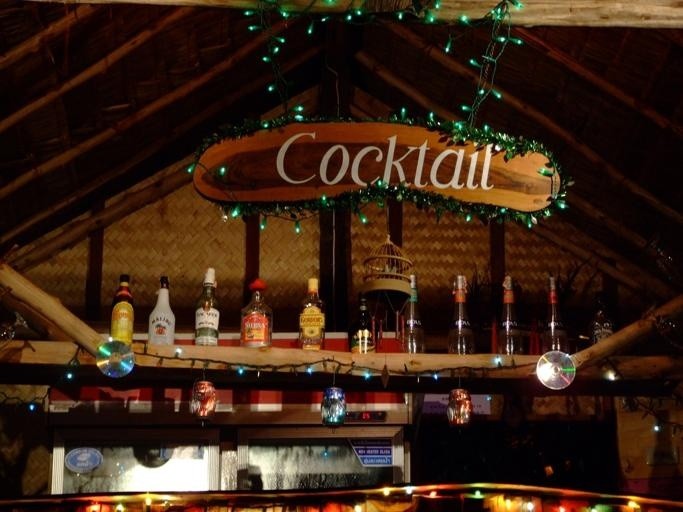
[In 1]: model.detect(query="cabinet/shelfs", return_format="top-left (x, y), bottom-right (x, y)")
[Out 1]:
top-left (0, 342), bottom-right (682, 382)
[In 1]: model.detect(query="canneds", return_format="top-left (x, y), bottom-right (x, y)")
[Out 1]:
top-left (447, 388), bottom-right (474, 427)
top-left (318, 387), bottom-right (347, 428)
top-left (190, 381), bottom-right (217, 416)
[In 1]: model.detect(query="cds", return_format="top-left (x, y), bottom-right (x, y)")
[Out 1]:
top-left (536, 351), bottom-right (576, 390)
top-left (95, 340), bottom-right (135, 379)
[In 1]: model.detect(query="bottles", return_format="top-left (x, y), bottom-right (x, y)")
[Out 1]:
top-left (498, 276), bottom-right (522, 357)
top-left (401, 273), bottom-right (426, 354)
top-left (147, 277), bottom-right (175, 348)
top-left (239, 278), bottom-right (273, 349)
top-left (446, 275), bottom-right (476, 355)
top-left (592, 293), bottom-right (614, 346)
top-left (545, 276), bottom-right (569, 352)
top-left (192, 267), bottom-right (221, 346)
top-left (348, 292), bottom-right (377, 354)
top-left (299, 277), bottom-right (326, 351)
top-left (110, 274), bottom-right (136, 344)
